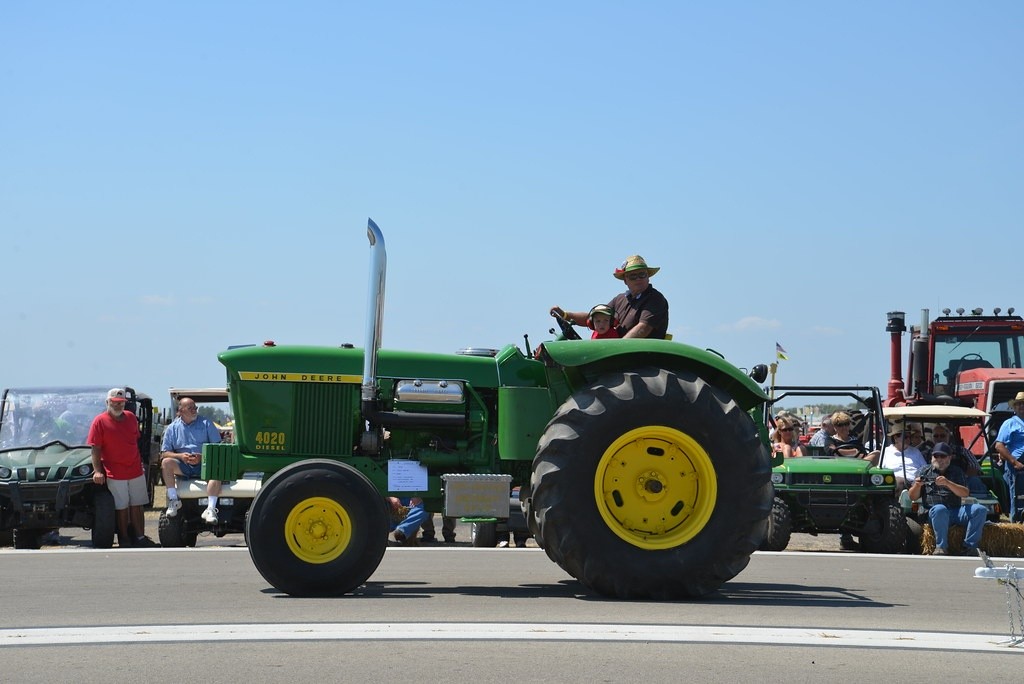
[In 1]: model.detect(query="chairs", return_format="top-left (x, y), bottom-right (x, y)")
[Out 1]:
top-left (949, 359), bottom-right (995, 396)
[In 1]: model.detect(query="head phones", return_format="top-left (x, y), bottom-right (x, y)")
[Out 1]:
top-left (585, 304), bottom-right (620, 330)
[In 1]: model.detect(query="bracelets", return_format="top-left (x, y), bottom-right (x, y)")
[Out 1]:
top-left (563, 311), bottom-right (567, 319)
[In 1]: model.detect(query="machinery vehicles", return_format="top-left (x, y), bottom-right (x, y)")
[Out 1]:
top-left (200, 216), bottom-right (777, 602)
top-left (882, 307), bottom-right (1024, 461)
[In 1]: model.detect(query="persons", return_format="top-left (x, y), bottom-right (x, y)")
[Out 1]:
top-left (386, 496), bottom-right (429, 545)
top-left (908, 442), bottom-right (986, 556)
top-left (923, 426), bottom-right (983, 477)
top-left (995, 392), bottom-right (1024, 524)
top-left (421, 511), bottom-right (456, 543)
top-left (87, 389), bottom-right (155, 546)
top-left (551, 255), bottom-right (668, 340)
top-left (823, 412), bottom-right (880, 466)
top-left (587, 304), bottom-right (619, 338)
top-left (809, 415), bottom-right (834, 447)
top-left (497, 531), bottom-right (530, 547)
top-left (770, 411), bottom-right (808, 458)
top-left (865, 421), bottom-right (929, 502)
top-left (160, 397), bottom-right (222, 525)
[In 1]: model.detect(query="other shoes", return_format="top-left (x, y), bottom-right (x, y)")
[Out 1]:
top-left (933, 549), bottom-right (948, 555)
top-left (516, 541), bottom-right (526, 547)
top-left (500, 540), bottom-right (509, 548)
top-left (420, 530), bottom-right (434, 542)
top-left (445, 537), bottom-right (455, 542)
top-left (387, 531), bottom-right (405, 546)
top-left (961, 547), bottom-right (979, 556)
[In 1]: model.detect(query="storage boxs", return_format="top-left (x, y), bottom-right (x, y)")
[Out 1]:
top-left (441, 473), bottom-right (512, 518)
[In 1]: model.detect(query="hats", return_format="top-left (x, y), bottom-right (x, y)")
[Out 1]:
top-left (613, 255), bottom-right (661, 280)
top-left (107, 388), bottom-right (128, 402)
top-left (591, 306), bottom-right (611, 316)
top-left (887, 423), bottom-right (915, 436)
top-left (1008, 392), bottom-right (1024, 408)
top-left (932, 442), bottom-right (953, 456)
top-left (909, 421), bottom-right (922, 434)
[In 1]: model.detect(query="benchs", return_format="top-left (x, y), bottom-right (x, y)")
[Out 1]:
top-left (806, 447), bottom-right (827, 456)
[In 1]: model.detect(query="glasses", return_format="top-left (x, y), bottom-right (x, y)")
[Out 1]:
top-left (834, 422), bottom-right (850, 427)
top-left (933, 434), bottom-right (945, 439)
top-left (180, 407), bottom-right (198, 411)
top-left (626, 272), bottom-right (648, 280)
top-left (933, 454), bottom-right (947, 458)
top-left (779, 427), bottom-right (793, 432)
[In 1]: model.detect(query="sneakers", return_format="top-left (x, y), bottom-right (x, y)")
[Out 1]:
top-left (119, 535), bottom-right (161, 547)
top-left (166, 499), bottom-right (182, 518)
top-left (200, 508), bottom-right (219, 525)
